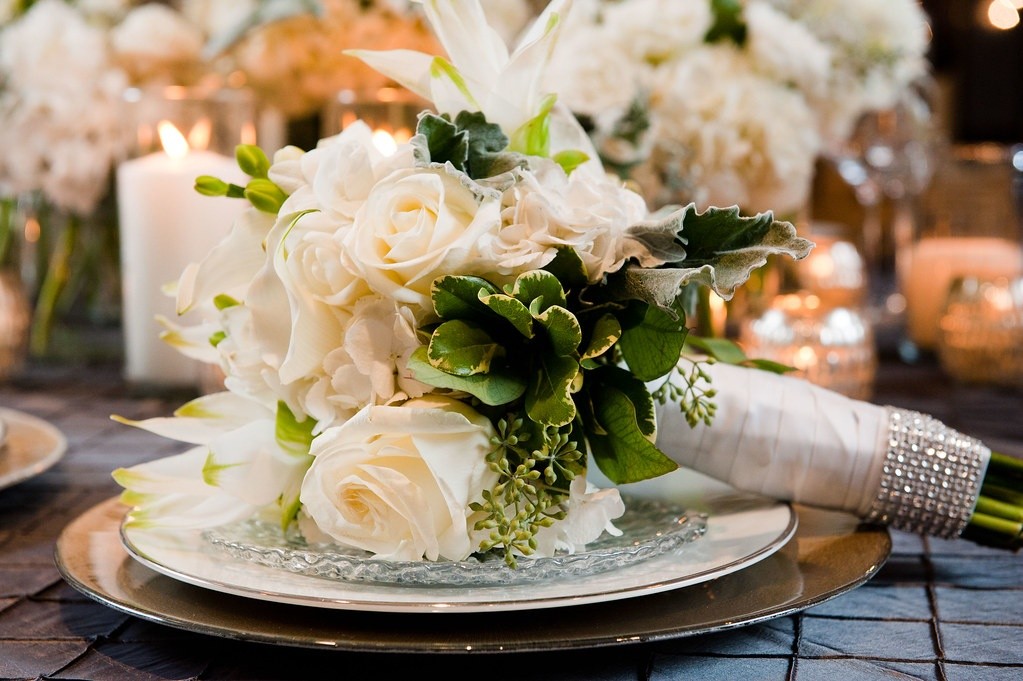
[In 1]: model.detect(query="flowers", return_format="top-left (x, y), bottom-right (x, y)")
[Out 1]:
top-left (107, 0), bottom-right (1023, 565)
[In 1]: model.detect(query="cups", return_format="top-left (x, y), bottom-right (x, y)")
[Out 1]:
top-left (124, 70), bottom-right (1022, 403)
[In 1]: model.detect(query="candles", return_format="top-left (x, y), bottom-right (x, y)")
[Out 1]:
top-left (117, 121), bottom-right (252, 385)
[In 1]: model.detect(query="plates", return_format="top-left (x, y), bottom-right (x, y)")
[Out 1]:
top-left (53, 480), bottom-right (894, 654)
top-left (2, 407), bottom-right (66, 493)
top-left (121, 452), bottom-right (796, 614)
